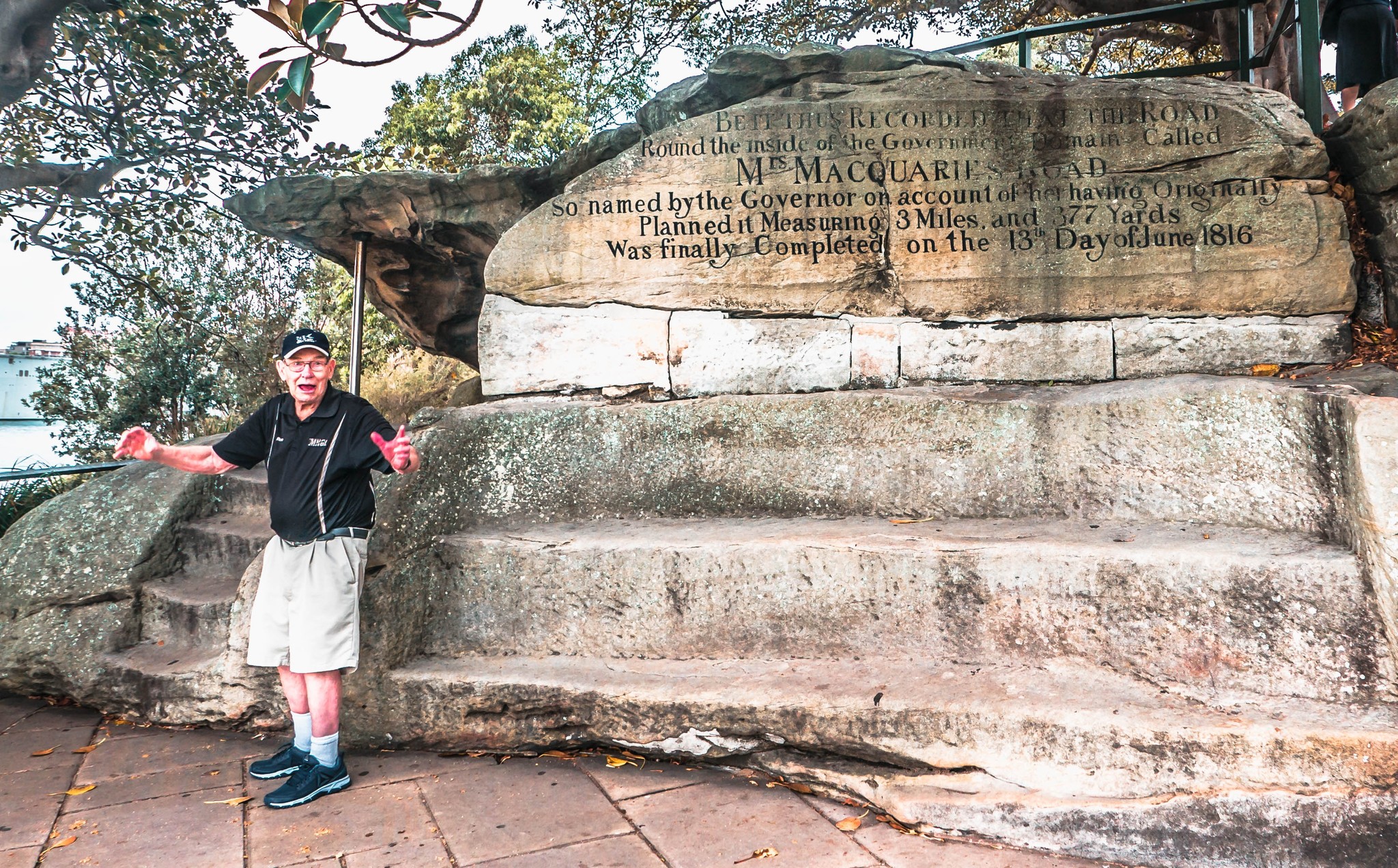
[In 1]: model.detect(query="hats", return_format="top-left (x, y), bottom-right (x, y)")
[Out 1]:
top-left (282, 328), bottom-right (330, 359)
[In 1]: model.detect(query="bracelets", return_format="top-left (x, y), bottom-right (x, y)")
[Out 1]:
top-left (401, 459), bottom-right (411, 470)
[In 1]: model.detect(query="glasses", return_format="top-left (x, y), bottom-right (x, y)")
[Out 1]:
top-left (284, 359), bottom-right (329, 372)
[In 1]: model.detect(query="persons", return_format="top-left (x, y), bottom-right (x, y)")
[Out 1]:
top-left (111, 328), bottom-right (420, 809)
top-left (1319, 0), bottom-right (1398, 113)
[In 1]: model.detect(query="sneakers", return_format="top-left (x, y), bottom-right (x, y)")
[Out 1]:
top-left (264, 755), bottom-right (351, 809)
top-left (249, 738), bottom-right (311, 779)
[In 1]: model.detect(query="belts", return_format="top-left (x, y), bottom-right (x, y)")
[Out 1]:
top-left (282, 527), bottom-right (368, 548)
